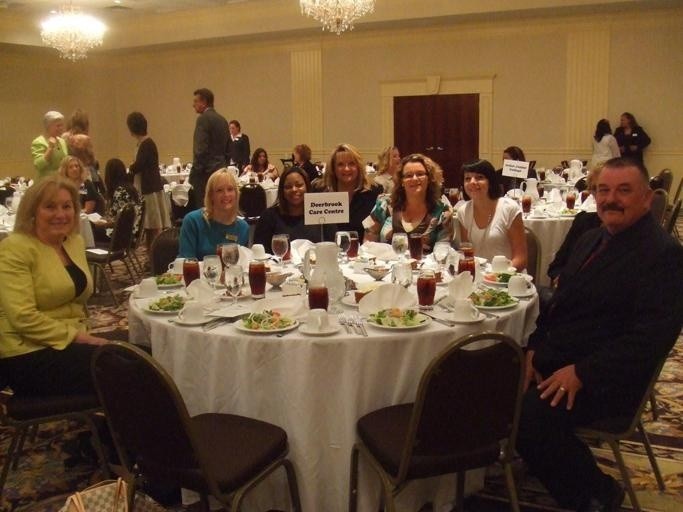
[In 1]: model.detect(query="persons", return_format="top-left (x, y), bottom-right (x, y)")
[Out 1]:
top-left (495, 146), bottom-right (539, 196)
top-left (515, 156), bottom-right (683, 512)
top-left (179, 168), bottom-right (249, 261)
top-left (363, 154), bottom-right (455, 254)
top-left (239, 148), bottom-right (280, 182)
top-left (68, 134), bottom-right (94, 181)
top-left (254, 166), bottom-right (330, 254)
top-left (57, 156), bottom-right (97, 214)
top-left (93, 158), bottom-right (143, 247)
top-left (188, 88), bottom-right (234, 211)
top-left (316, 143), bottom-right (384, 246)
top-left (1, 176), bottom-right (117, 395)
top-left (126, 111), bottom-right (172, 275)
top-left (590, 118), bottom-right (621, 173)
top-left (60, 108), bottom-right (88, 143)
top-left (294, 144), bottom-right (319, 182)
top-left (613, 112), bottom-right (651, 167)
top-left (228, 120), bottom-right (250, 177)
top-left (31, 111), bottom-right (68, 184)
top-left (453, 159), bottom-right (528, 273)
top-left (371, 146), bottom-right (400, 194)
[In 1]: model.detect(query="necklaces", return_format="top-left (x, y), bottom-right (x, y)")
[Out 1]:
top-left (465, 199), bottom-right (493, 258)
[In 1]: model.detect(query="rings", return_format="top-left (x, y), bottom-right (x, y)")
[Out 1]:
top-left (559, 385), bottom-right (567, 394)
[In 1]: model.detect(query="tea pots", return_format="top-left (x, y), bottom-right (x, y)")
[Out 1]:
top-left (304, 242), bottom-right (345, 304)
top-left (520, 178), bottom-right (539, 200)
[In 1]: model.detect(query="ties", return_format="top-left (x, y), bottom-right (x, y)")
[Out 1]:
top-left (572, 234), bottom-right (615, 283)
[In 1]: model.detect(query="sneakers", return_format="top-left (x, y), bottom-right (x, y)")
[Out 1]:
top-left (589, 475), bottom-right (625, 511)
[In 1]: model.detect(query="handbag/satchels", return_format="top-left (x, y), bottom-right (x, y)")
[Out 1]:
top-left (59, 477), bottom-right (130, 512)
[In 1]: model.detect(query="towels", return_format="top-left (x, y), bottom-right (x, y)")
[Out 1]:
top-left (359, 284), bottom-right (418, 314)
top-left (447, 271), bottom-right (474, 307)
top-left (186, 280), bottom-right (215, 305)
top-left (360, 242), bottom-right (394, 260)
top-left (236, 244), bottom-right (250, 269)
top-left (289, 240), bottom-right (314, 263)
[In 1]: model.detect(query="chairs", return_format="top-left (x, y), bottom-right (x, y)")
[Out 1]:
top-left (0, 364), bottom-right (100, 502)
top-left (651, 188), bottom-right (669, 231)
top-left (650, 176), bottom-right (664, 189)
top-left (150, 227), bottom-right (182, 277)
top-left (88, 342), bottom-right (300, 512)
top-left (169, 187), bottom-right (195, 225)
top-left (575, 290), bottom-right (683, 511)
top-left (85, 204), bottom-right (136, 305)
top-left (673, 177), bottom-right (683, 204)
top-left (237, 183), bottom-right (267, 225)
top-left (94, 200), bottom-right (147, 279)
top-left (658, 168), bottom-right (673, 196)
top-left (525, 224), bottom-right (542, 294)
top-left (663, 196), bottom-right (683, 236)
top-left (348, 332), bottom-right (525, 510)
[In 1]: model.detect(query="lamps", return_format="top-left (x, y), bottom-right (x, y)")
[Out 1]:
top-left (38, 7), bottom-right (107, 61)
top-left (298, 0), bottom-right (376, 37)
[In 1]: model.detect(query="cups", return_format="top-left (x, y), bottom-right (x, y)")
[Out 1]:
top-left (168, 258), bottom-right (185, 273)
top-left (204, 255), bottom-right (221, 291)
top-left (248, 262), bottom-right (266, 299)
top-left (459, 242), bottom-right (474, 258)
top-left (347, 231), bottom-right (359, 260)
top-left (392, 263), bottom-right (413, 287)
top-left (492, 256), bottom-right (512, 271)
top-left (566, 192), bottom-right (576, 209)
top-left (217, 243), bottom-right (226, 284)
top-left (335, 232), bottom-right (350, 264)
top-left (408, 233), bottom-right (424, 260)
top-left (282, 234), bottom-right (290, 261)
top-left (417, 269), bottom-right (436, 306)
top-left (222, 244), bottom-right (240, 267)
top-left (133, 279), bottom-right (156, 296)
top-left (521, 196), bottom-right (531, 213)
top-left (272, 235), bottom-right (288, 264)
top-left (225, 265), bottom-right (244, 309)
top-left (458, 255), bottom-right (475, 283)
top-left (455, 300), bottom-right (479, 319)
top-left (251, 244), bottom-right (265, 257)
top-left (581, 192), bottom-right (590, 204)
top-left (508, 276), bottom-right (528, 295)
top-left (538, 186), bottom-right (544, 197)
top-left (309, 269), bottom-right (329, 312)
top-left (308, 309), bottom-right (329, 332)
top-left (183, 257), bottom-right (200, 288)
top-left (392, 234), bottom-right (408, 254)
top-left (433, 242), bottom-right (449, 268)
top-left (178, 301), bottom-right (204, 322)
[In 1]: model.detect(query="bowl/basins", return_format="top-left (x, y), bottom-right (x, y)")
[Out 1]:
top-left (364, 267), bottom-right (395, 281)
top-left (266, 273), bottom-right (293, 291)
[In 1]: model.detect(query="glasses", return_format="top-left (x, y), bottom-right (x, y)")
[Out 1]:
top-left (401, 172), bottom-right (428, 179)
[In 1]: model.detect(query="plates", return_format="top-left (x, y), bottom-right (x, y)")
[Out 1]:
top-left (475, 256), bottom-right (488, 264)
top-left (486, 267), bottom-right (517, 275)
top-left (158, 281), bottom-right (182, 289)
top-left (367, 314), bottom-right (432, 329)
top-left (500, 288), bottom-right (536, 297)
top-left (436, 277), bottom-right (453, 287)
top-left (175, 315), bottom-right (221, 326)
top-left (299, 324), bottom-right (341, 334)
top-left (216, 290), bottom-right (248, 301)
top-left (135, 290), bottom-right (188, 315)
top-left (205, 304), bottom-right (251, 317)
top-left (447, 313), bottom-right (486, 325)
top-left (561, 214), bottom-right (576, 217)
top-left (481, 273), bottom-right (533, 285)
top-left (341, 295), bottom-right (359, 307)
top-left (473, 296), bottom-right (519, 310)
top-left (234, 318), bottom-right (299, 333)
top-left (531, 215), bottom-right (548, 219)
